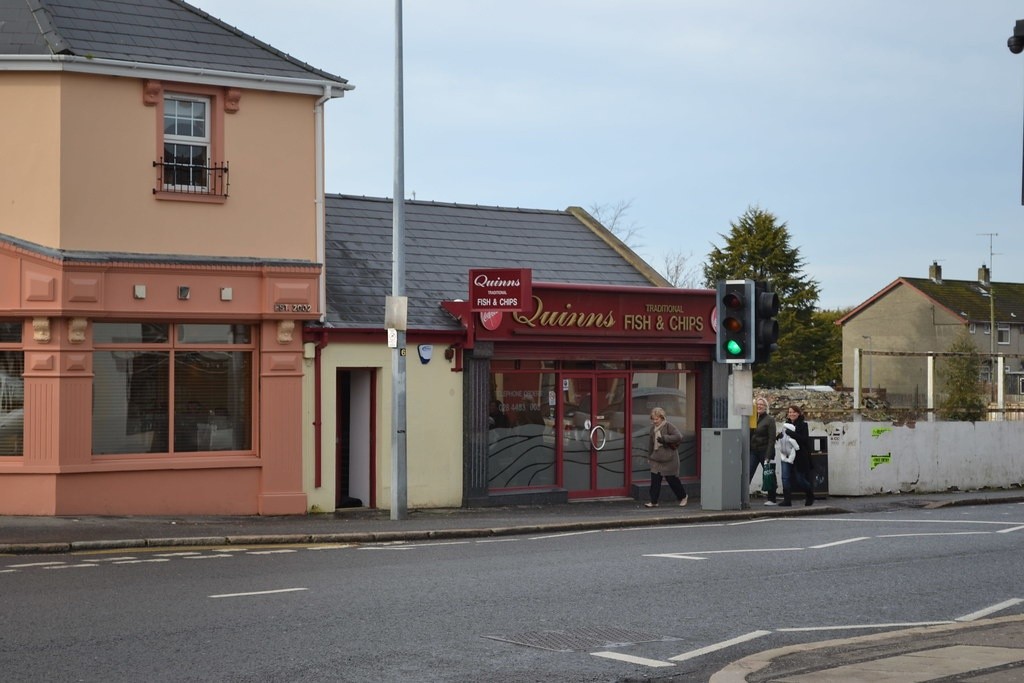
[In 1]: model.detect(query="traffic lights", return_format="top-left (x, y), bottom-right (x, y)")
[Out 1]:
top-left (755, 281), bottom-right (780, 363)
top-left (716, 281), bottom-right (755, 364)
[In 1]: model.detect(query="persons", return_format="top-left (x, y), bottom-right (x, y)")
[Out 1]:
top-left (751, 397), bottom-right (779, 506)
top-left (488, 399), bottom-right (509, 454)
top-left (777, 406), bottom-right (815, 506)
top-left (644, 407), bottom-right (688, 506)
top-left (517, 397), bottom-right (545, 484)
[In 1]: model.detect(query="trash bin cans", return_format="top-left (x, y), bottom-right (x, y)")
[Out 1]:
top-left (790, 436), bottom-right (829, 493)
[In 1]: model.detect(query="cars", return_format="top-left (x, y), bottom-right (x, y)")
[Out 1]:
top-left (543, 385), bottom-right (686, 455)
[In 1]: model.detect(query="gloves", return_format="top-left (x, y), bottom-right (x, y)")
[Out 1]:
top-left (776, 432), bottom-right (783, 440)
top-left (785, 429), bottom-right (792, 435)
top-left (657, 436), bottom-right (664, 443)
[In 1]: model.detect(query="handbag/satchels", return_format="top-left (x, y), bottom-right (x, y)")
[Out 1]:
top-left (761, 459), bottom-right (778, 491)
top-left (748, 462), bottom-right (768, 494)
top-left (650, 445), bottom-right (674, 462)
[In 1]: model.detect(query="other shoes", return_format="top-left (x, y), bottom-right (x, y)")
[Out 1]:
top-left (644, 502), bottom-right (659, 507)
top-left (679, 493), bottom-right (688, 506)
top-left (778, 500), bottom-right (792, 506)
top-left (764, 501), bottom-right (776, 506)
top-left (805, 495), bottom-right (814, 506)
